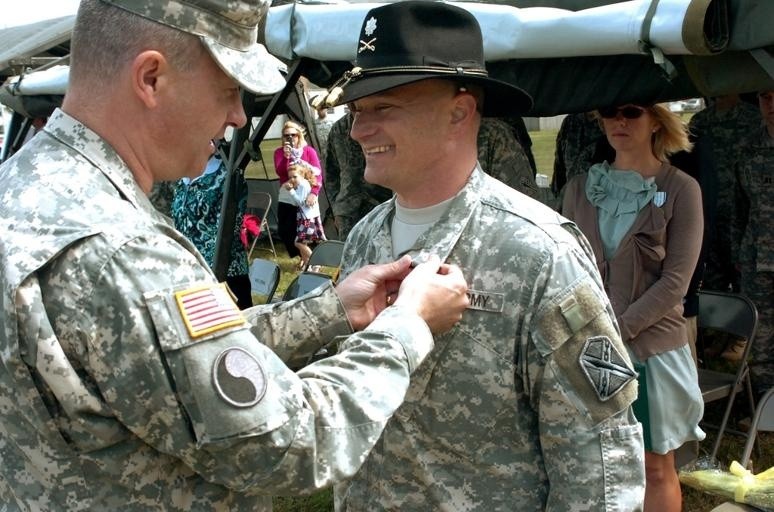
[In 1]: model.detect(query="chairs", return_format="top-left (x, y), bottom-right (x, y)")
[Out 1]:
top-left (687, 283), bottom-right (763, 478)
top-left (232, 188), bottom-right (345, 371)
top-left (712, 386), bottom-right (773, 512)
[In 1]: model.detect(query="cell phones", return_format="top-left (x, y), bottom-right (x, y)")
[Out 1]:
top-left (286, 137), bottom-right (293, 148)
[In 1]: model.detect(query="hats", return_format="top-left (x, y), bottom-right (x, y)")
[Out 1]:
top-left (307, 0), bottom-right (536, 117)
top-left (96, 1), bottom-right (288, 96)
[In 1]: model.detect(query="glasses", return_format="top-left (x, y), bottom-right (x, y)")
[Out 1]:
top-left (600, 106), bottom-right (646, 120)
top-left (285, 133), bottom-right (298, 137)
